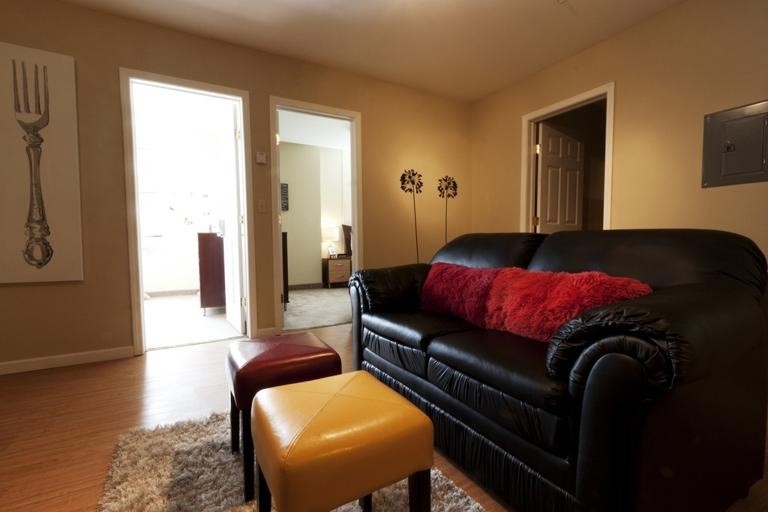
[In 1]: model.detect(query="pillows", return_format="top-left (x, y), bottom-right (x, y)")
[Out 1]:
top-left (488, 267), bottom-right (650, 345)
top-left (422, 261), bottom-right (496, 324)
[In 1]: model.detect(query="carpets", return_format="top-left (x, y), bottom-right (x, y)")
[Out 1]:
top-left (99, 415), bottom-right (480, 512)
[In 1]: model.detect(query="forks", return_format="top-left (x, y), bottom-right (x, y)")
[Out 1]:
top-left (13, 60), bottom-right (55, 268)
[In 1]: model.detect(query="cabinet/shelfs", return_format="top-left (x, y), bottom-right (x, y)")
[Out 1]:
top-left (322, 258), bottom-right (351, 288)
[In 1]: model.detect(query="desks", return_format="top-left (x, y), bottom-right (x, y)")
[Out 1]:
top-left (282, 232), bottom-right (289, 311)
top-left (198, 233), bottom-right (225, 317)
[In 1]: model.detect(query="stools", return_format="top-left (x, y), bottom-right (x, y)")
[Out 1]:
top-left (225, 331), bottom-right (342, 502)
top-left (249, 371), bottom-right (433, 512)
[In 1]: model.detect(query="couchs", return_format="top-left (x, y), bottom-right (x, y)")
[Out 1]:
top-left (349, 233), bottom-right (767, 511)
